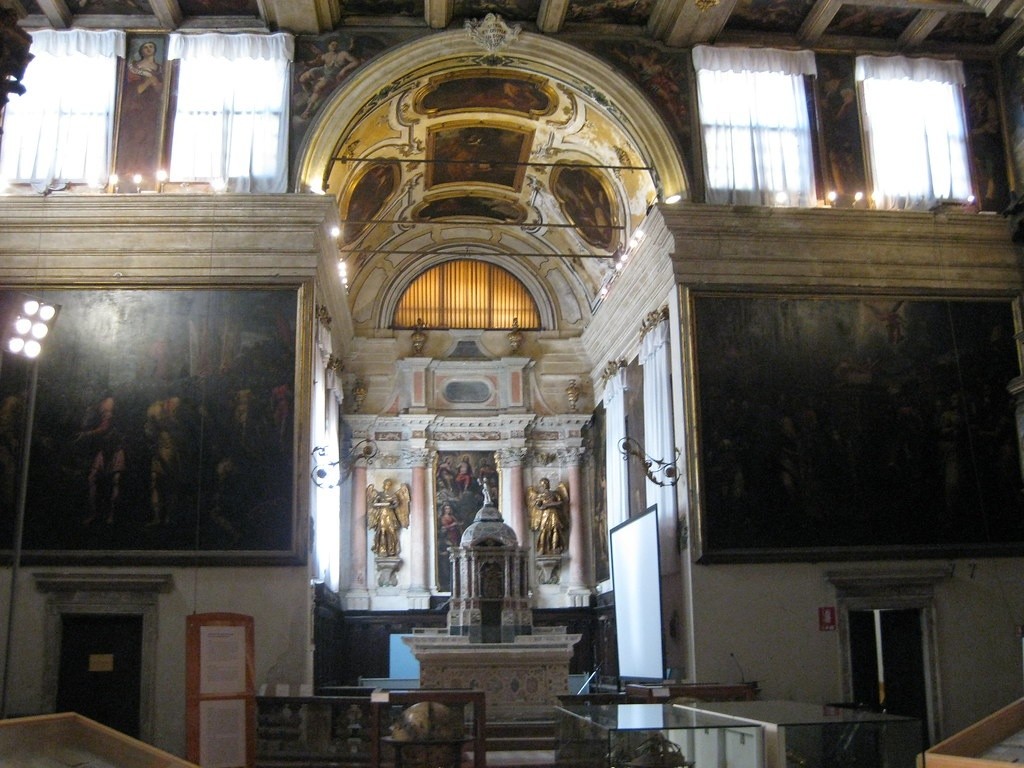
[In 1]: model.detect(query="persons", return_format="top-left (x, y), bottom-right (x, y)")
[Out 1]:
top-left (526, 478), bottom-right (570, 556)
top-left (366, 479), bottom-right (412, 557)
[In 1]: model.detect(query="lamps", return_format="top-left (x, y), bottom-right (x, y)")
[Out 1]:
top-left (0, 292), bottom-right (62, 363)
top-left (109, 174), bottom-right (122, 193)
top-left (133, 174), bottom-right (146, 193)
top-left (157, 170), bottom-right (169, 193)
